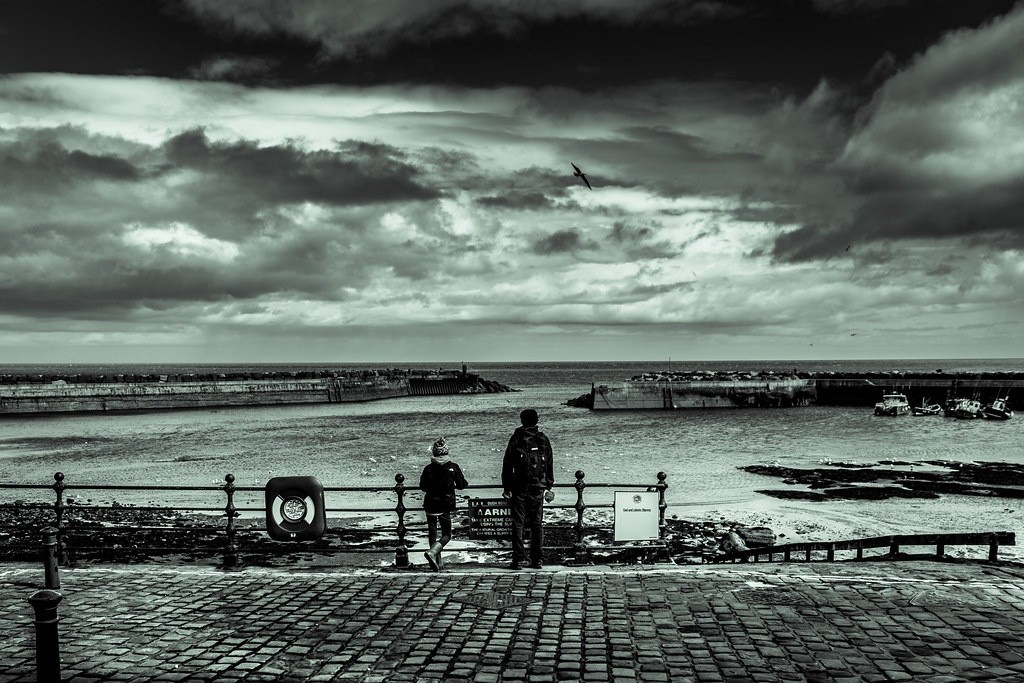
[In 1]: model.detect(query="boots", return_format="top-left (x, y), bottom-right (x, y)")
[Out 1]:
top-left (424, 541), bottom-right (443, 571)
top-left (436, 553), bottom-right (444, 570)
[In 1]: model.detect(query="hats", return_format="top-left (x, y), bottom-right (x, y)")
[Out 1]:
top-left (432, 437), bottom-right (449, 457)
top-left (520, 409), bottom-right (539, 427)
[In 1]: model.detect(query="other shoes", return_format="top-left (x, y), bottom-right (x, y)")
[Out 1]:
top-left (510, 562), bottom-right (522, 570)
top-left (528, 561), bottom-right (542, 569)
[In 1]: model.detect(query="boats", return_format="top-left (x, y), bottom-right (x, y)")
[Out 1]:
top-left (954, 388), bottom-right (983, 419)
top-left (873, 392), bottom-right (911, 416)
top-left (985, 394), bottom-right (1014, 420)
top-left (944, 397), bottom-right (967, 417)
top-left (911, 404), bottom-right (941, 415)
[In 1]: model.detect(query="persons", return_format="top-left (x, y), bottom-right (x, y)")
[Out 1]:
top-left (501, 408), bottom-right (555, 569)
top-left (420, 438), bottom-right (469, 571)
